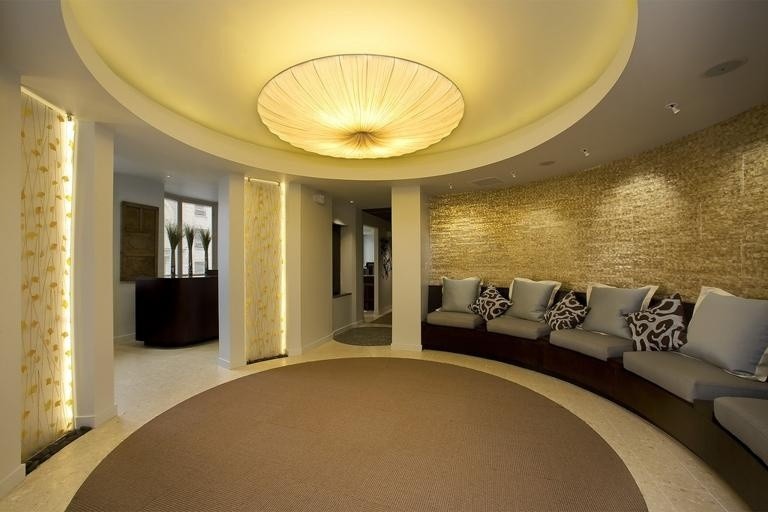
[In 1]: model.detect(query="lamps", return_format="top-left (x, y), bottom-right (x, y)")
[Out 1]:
top-left (256, 54), bottom-right (466, 161)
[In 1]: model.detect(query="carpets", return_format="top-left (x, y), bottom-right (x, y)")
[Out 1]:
top-left (333, 325), bottom-right (392, 347)
top-left (63, 356), bottom-right (651, 512)
